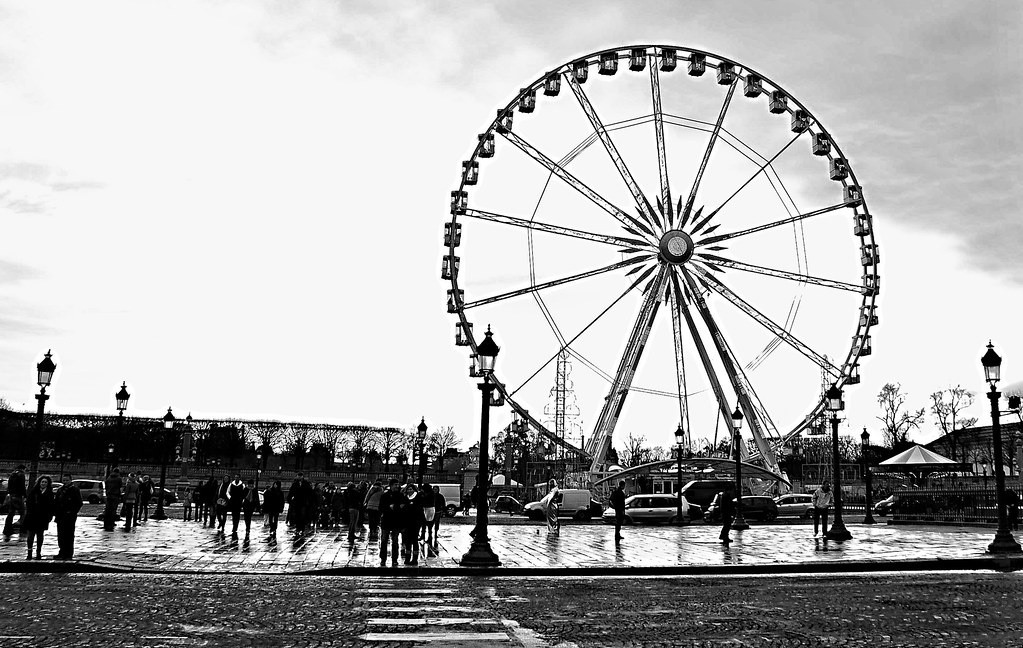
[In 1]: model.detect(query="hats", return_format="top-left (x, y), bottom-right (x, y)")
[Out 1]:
top-left (128, 472), bottom-right (136, 481)
top-left (422, 482), bottom-right (432, 490)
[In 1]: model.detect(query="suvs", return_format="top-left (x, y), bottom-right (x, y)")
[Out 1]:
top-left (53, 480), bottom-right (105, 504)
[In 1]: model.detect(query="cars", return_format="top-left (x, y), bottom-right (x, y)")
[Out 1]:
top-left (875, 495), bottom-right (894, 515)
top-left (493, 496), bottom-right (523, 515)
top-left (602, 493), bottom-right (687, 525)
top-left (0, 479), bottom-right (29, 510)
top-left (704, 496), bottom-right (779, 525)
top-left (121, 487), bottom-right (179, 506)
top-left (258, 492), bottom-right (264, 514)
top-left (773, 494), bottom-right (815, 519)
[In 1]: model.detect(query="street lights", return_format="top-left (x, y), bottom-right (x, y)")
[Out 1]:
top-left (460, 324), bottom-right (503, 566)
top-left (149, 406), bottom-right (175, 519)
top-left (417, 415), bottom-right (428, 489)
top-left (114, 379), bottom-right (130, 469)
top-left (675, 422), bottom-right (685, 525)
top-left (255, 450), bottom-right (264, 491)
top-left (28, 348), bottom-right (57, 502)
top-left (827, 382), bottom-right (852, 539)
top-left (106, 443), bottom-right (114, 481)
top-left (732, 404), bottom-right (751, 530)
top-left (980, 340), bottom-right (1023, 555)
top-left (861, 427), bottom-right (876, 524)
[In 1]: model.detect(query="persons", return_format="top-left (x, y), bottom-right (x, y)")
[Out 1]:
top-left (184, 474), bottom-right (491, 566)
top-left (719, 485), bottom-right (734, 542)
top-left (811, 480), bottom-right (834, 538)
top-left (1006, 489), bottom-right (1021, 531)
top-left (2, 465), bottom-right (155, 560)
top-left (611, 481), bottom-right (624, 539)
top-left (545, 479), bottom-right (559, 534)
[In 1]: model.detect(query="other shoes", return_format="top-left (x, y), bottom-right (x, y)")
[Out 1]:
top-left (288, 523), bottom-right (349, 530)
top-left (26, 553), bottom-right (72, 560)
top-left (469, 533), bottom-right (491, 541)
top-left (392, 559), bottom-right (398, 566)
top-left (269, 528), bottom-right (276, 536)
top-left (294, 532), bottom-right (305, 540)
top-left (404, 559), bottom-right (418, 565)
top-left (184, 517), bottom-right (202, 522)
top-left (123, 517), bottom-right (147, 526)
top-left (418, 534), bottom-right (439, 541)
top-left (615, 536), bottom-right (624, 539)
top-left (719, 535), bottom-right (733, 542)
top-left (348, 533), bottom-right (378, 539)
top-left (203, 520), bottom-right (250, 533)
top-left (813, 532), bottom-right (826, 536)
top-left (381, 559), bottom-right (385, 565)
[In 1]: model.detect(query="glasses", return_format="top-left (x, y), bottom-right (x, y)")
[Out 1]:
top-left (235, 477), bottom-right (239, 478)
top-left (248, 486), bottom-right (254, 488)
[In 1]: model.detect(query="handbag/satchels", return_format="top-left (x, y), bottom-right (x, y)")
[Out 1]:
top-left (2, 493), bottom-right (19, 508)
top-left (217, 498), bottom-right (227, 506)
top-left (264, 513), bottom-right (274, 529)
top-left (423, 507), bottom-right (436, 521)
top-left (361, 502), bottom-right (368, 513)
top-left (149, 481), bottom-right (155, 496)
top-left (121, 503), bottom-right (129, 517)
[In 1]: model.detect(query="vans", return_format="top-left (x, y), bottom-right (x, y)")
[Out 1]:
top-left (675, 479), bottom-right (736, 509)
top-left (402, 483), bottom-right (461, 517)
top-left (524, 489), bottom-right (592, 521)
